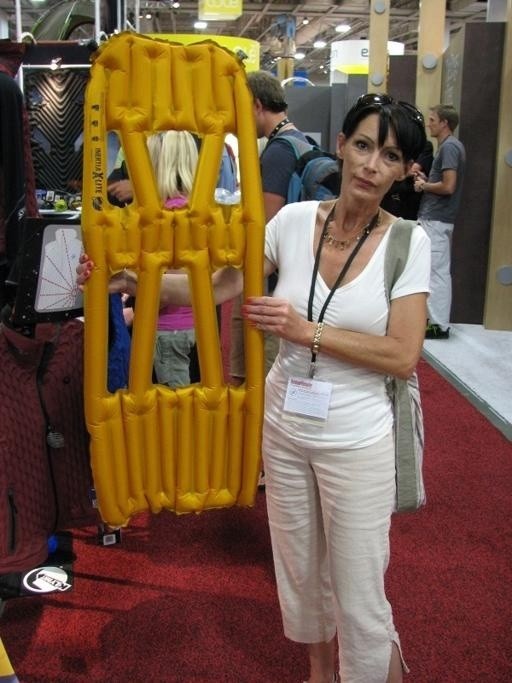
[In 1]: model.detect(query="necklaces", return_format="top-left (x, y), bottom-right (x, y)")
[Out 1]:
top-left (322, 195), bottom-right (382, 250)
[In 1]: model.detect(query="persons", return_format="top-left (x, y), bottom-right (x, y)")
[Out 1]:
top-left (411, 103), bottom-right (467, 340)
top-left (108, 68), bottom-right (323, 490)
top-left (76, 91), bottom-right (435, 682)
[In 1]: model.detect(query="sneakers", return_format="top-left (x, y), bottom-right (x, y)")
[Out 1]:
top-left (426, 324), bottom-right (450, 339)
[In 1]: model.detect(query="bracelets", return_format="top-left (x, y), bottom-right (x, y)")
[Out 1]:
top-left (312, 321), bottom-right (323, 354)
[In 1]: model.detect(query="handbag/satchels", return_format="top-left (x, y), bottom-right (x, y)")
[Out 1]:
top-left (383, 376), bottom-right (425, 512)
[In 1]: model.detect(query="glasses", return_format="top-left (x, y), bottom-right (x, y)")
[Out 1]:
top-left (357, 93), bottom-right (424, 131)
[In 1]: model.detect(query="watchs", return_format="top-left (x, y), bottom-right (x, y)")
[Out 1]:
top-left (419, 182), bottom-right (425, 189)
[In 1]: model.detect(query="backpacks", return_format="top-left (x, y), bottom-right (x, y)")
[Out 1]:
top-left (270, 136), bottom-right (340, 203)
